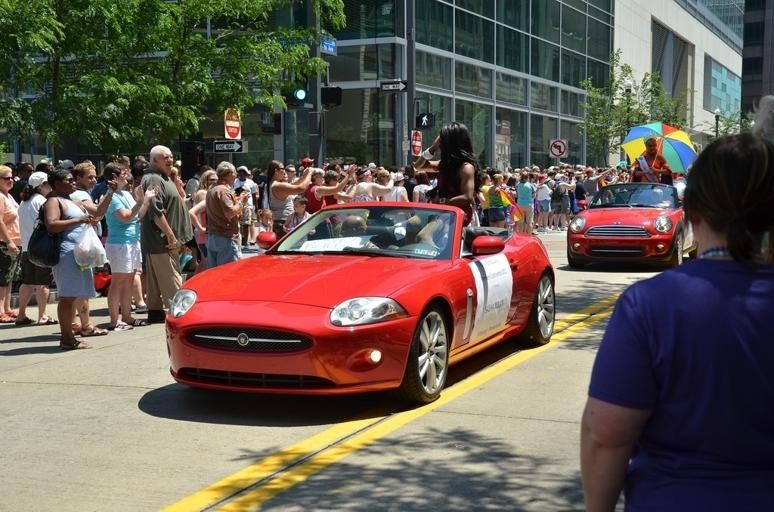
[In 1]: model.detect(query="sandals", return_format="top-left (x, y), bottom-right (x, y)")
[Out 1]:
top-left (0, 311), bottom-right (58, 325)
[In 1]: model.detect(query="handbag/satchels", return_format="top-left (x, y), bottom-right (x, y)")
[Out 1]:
top-left (27, 219), bottom-right (59, 269)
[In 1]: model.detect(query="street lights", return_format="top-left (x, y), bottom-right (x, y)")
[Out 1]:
top-left (626, 84), bottom-right (632, 165)
top-left (714, 109), bottom-right (721, 139)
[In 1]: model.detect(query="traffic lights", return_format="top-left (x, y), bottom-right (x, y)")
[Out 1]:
top-left (420, 115), bottom-right (432, 126)
top-left (294, 90), bottom-right (306, 100)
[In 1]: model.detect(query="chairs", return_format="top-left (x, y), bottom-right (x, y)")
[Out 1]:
top-left (366, 226), bottom-right (395, 250)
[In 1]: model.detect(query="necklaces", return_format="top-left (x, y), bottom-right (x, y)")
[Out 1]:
top-left (699, 246), bottom-right (740, 257)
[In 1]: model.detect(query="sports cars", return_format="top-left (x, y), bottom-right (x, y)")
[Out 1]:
top-left (567, 183), bottom-right (697, 269)
top-left (165, 201), bottom-right (556, 402)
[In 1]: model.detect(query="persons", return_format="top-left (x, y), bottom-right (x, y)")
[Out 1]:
top-left (581, 135), bottom-right (770, 512)
top-left (632, 138), bottom-right (670, 185)
top-left (44, 169), bottom-right (119, 350)
top-left (404, 122), bottom-right (483, 254)
top-left (340, 215), bottom-right (381, 251)
top-left (472, 164), bottom-right (634, 236)
top-left (0, 144), bottom-right (443, 334)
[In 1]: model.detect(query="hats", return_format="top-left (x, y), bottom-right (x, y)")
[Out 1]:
top-left (586, 169), bottom-right (594, 172)
top-left (28, 171), bottom-right (48, 189)
top-left (537, 175), bottom-right (546, 181)
top-left (301, 158), bottom-right (315, 168)
top-left (237, 166), bottom-right (251, 175)
top-left (61, 160), bottom-right (75, 170)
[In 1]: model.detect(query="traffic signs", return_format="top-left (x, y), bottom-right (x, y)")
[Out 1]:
top-left (381, 82), bottom-right (406, 91)
top-left (214, 141), bottom-right (243, 153)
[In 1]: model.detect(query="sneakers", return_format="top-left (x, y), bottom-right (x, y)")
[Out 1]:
top-left (108, 303), bottom-right (166, 330)
top-left (532, 226), bottom-right (568, 235)
top-left (60, 322), bottom-right (109, 350)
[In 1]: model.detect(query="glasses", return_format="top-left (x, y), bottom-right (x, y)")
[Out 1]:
top-left (0, 177), bottom-right (13, 180)
top-left (66, 178), bottom-right (76, 183)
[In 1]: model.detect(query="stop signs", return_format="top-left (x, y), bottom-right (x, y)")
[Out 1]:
top-left (412, 131), bottom-right (422, 156)
top-left (226, 109), bottom-right (240, 138)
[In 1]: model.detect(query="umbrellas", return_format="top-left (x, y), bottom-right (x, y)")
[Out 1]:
top-left (622, 120), bottom-right (697, 171)
